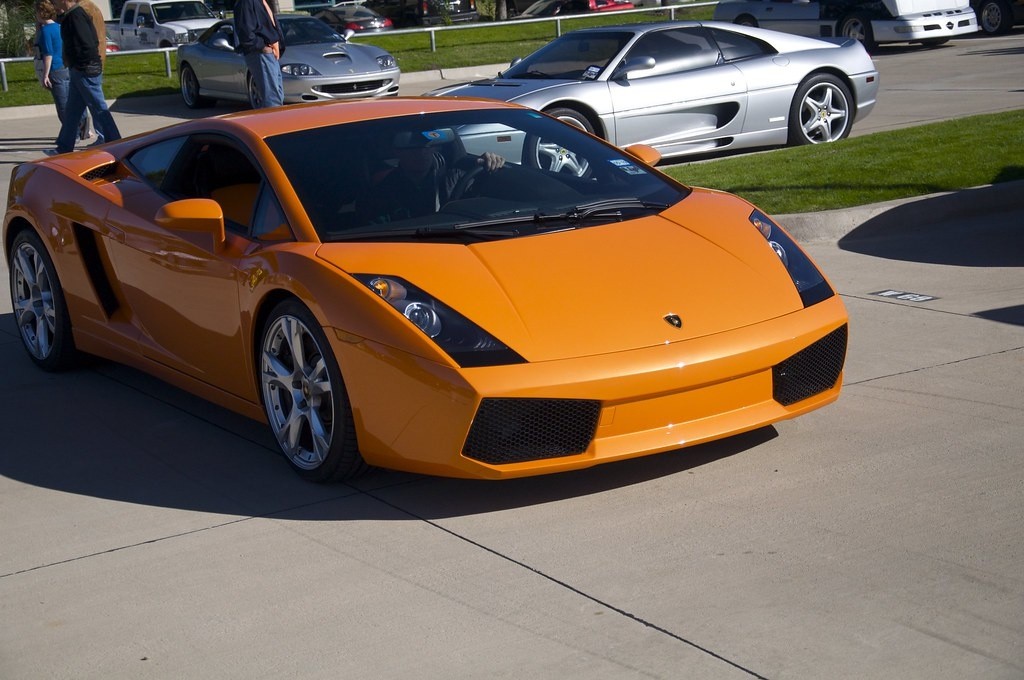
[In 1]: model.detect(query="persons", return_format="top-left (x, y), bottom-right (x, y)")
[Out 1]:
top-left (376, 145), bottom-right (506, 215)
top-left (234, 0), bottom-right (286, 111)
top-left (28, 0), bottom-right (121, 156)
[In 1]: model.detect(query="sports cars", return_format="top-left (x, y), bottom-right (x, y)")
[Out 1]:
top-left (421, 18), bottom-right (880, 180)
top-left (3, 96), bottom-right (848, 483)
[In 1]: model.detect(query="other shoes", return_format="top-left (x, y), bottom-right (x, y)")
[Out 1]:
top-left (87, 138), bottom-right (105, 146)
top-left (42, 150), bottom-right (59, 156)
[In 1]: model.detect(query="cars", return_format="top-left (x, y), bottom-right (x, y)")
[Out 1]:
top-left (540, 0), bottom-right (634, 16)
top-left (177, 13), bottom-right (401, 111)
top-left (294, 0), bottom-right (480, 34)
top-left (712, 0), bottom-right (977, 55)
top-left (968, 0), bottom-right (1023, 36)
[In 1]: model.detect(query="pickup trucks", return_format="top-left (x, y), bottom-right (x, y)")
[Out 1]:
top-left (102, 0), bottom-right (234, 51)
